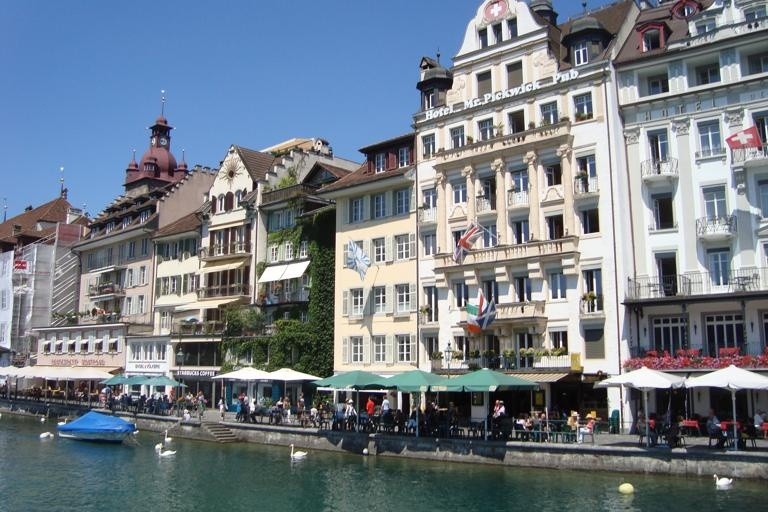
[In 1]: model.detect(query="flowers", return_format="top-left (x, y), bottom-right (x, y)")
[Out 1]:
top-left (622, 353), bottom-right (768, 368)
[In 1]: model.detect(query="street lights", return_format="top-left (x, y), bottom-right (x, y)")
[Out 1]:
top-left (176, 348), bottom-right (185, 417)
top-left (443, 341), bottom-right (455, 378)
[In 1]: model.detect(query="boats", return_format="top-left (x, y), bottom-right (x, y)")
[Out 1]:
top-left (58, 411), bottom-right (134, 444)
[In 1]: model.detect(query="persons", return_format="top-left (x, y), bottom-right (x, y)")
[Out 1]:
top-left (1, 382), bottom-right (598, 451)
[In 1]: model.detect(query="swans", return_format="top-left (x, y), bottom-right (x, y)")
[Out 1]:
top-left (57, 418), bottom-right (68, 425)
top-left (712, 473), bottom-right (734, 485)
top-left (155, 442), bottom-right (179, 458)
top-left (288, 443), bottom-right (309, 457)
top-left (44, 429), bottom-right (72, 440)
top-left (39, 431), bottom-right (54, 438)
top-left (162, 429), bottom-right (172, 442)
top-left (133, 423), bottom-right (139, 435)
top-left (40, 414), bottom-right (45, 423)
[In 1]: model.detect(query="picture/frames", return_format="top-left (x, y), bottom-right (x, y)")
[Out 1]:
top-left (472, 391), bottom-right (484, 405)
top-left (531, 390), bottom-right (545, 407)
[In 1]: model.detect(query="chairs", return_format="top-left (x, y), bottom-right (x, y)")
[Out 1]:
top-left (319, 409), bottom-right (595, 444)
top-left (634, 422), bottom-right (758, 448)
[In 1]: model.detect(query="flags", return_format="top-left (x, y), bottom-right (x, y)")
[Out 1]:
top-left (725, 125), bottom-right (762, 154)
top-left (463, 288), bottom-right (498, 334)
top-left (344, 239), bottom-right (371, 279)
top-left (451, 222), bottom-right (486, 268)
